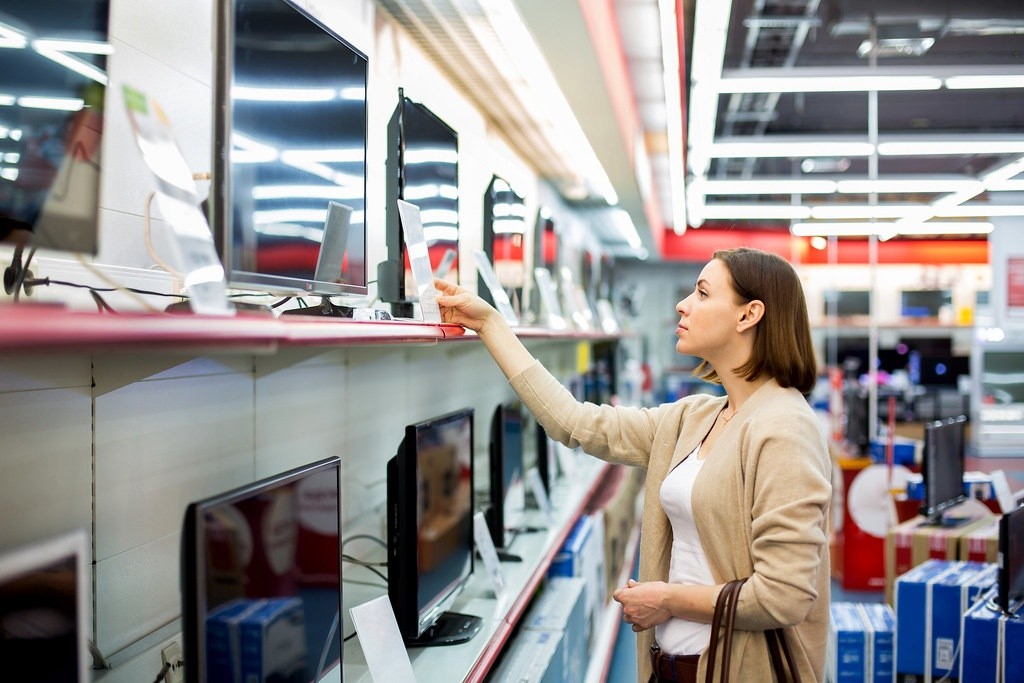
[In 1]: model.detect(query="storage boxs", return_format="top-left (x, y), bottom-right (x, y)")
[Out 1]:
top-left (210, 594), bottom-right (312, 683)
top-left (479, 440), bottom-right (1024, 683)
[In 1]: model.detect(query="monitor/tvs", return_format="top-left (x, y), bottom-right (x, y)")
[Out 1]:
top-left (919, 414), bottom-right (971, 527)
top-left (0, 366), bottom-right (612, 683)
top-left (0, 0), bottom-right (603, 325)
top-left (987, 504), bottom-right (1024, 619)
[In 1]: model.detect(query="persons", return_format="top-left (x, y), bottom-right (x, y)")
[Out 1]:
top-left (432, 248), bottom-right (834, 683)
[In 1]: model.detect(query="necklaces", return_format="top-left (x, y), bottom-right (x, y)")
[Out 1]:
top-left (721, 409), bottom-right (737, 426)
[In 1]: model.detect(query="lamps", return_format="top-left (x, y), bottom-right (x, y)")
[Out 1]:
top-left (677, 0), bottom-right (1024, 245)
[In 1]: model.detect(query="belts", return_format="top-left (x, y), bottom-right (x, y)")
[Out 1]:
top-left (650, 648), bottom-right (700, 683)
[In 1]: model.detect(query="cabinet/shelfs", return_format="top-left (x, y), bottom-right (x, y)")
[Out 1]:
top-left (0, 0), bottom-right (632, 683)
